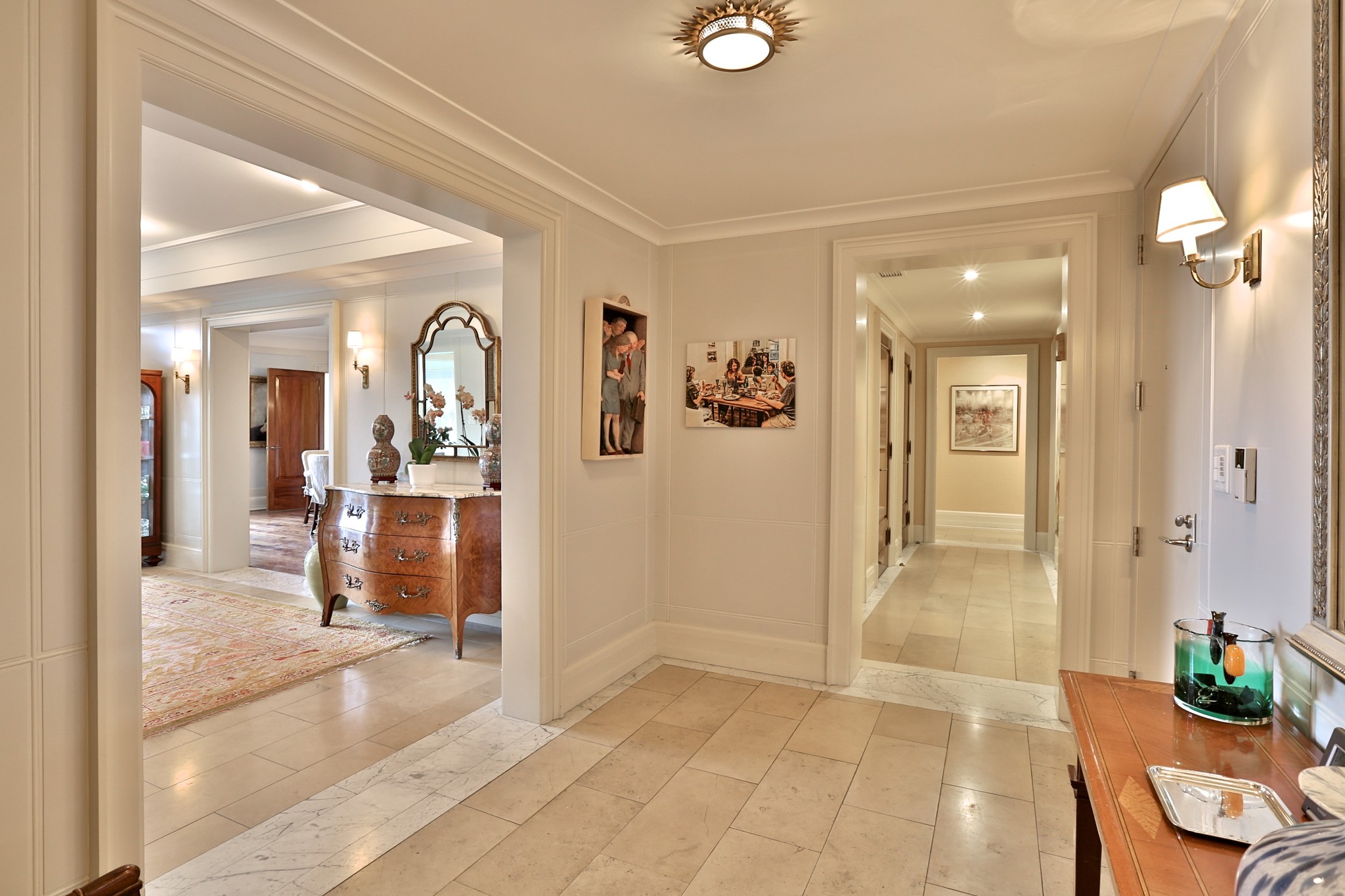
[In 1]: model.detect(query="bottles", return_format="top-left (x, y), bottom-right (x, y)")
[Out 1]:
top-left (733, 376), bottom-right (739, 394)
top-left (750, 383), bottom-right (755, 397)
top-left (755, 385), bottom-right (758, 395)
top-left (760, 379), bottom-right (767, 392)
top-left (744, 376), bottom-right (748, 388)
top-left (1172, 618), bottom-right (1275, 725)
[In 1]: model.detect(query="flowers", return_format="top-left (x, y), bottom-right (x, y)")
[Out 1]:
top-left (456, 383), bottom-right (486, 458)
top-left (402, 381), bottom-right (447, 475)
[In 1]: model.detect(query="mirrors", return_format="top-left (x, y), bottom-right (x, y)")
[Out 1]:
top-left (409, 302), bottom-right (501, 463)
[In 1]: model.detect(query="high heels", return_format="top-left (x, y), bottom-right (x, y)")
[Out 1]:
top-left (614, 445), bottom-right (626, 454)
top-left (604, 443), bottom-right (619, 455)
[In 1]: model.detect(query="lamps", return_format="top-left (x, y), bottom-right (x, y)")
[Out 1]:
top-left (347, 330), bottom-right (370, 389)
top-left (170, 346), bottom-right (191, 394)
top-left (1155, 175), bottom-right (1262, 291)
top-left (672, 0), bottom-right (800, 73)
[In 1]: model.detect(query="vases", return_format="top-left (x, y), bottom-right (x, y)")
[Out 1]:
top-left (407, 464), bottom-right (437, 488)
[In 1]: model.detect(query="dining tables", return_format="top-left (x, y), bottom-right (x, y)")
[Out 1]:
top-left (695, 384), bottom-right (785, 427)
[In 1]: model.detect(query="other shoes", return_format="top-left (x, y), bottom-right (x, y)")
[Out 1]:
top-left (622, 447), bottom-right (631, 454)
top-left (718, 416), bottom-right (728, 425)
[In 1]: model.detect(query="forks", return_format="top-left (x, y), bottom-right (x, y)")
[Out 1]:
top-left (768, 375), bottom-right (776, 388)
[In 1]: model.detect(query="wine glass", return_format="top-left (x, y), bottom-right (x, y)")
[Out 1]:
top-left (723, 382), bottom-right (726, 394)
top-left (716, 379), bottom-right (719, 389)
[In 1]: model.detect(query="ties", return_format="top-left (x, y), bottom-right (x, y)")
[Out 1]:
top-left (626, 353), bottom-right (631, 375)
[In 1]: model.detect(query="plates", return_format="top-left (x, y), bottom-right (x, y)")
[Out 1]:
top-left (763, 393), bottom-right (782, 401)
top-left (723, 394), bottom-right (740, 400)
top-left (745, 391), bottom-right (764, 398)
top-left (700, 392), bottom-right (712, 396)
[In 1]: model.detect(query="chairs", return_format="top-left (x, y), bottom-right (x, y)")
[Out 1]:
top-left (307, 453), bottom-right (330, 535)
top-left (301, 449), bottom-right (329, 525)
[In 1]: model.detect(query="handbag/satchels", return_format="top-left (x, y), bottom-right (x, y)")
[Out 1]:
top-left (631, 396), bottom-right (646, 425)
top-left (619, 399), bottom-right (625, 422)
top-left (618, 360), bottom-right (625, 374)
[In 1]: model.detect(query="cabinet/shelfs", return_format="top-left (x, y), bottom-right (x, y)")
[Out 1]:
top-left (139, 369), bottom-right (164, 567)
top-left (318, 483), bottom-right (502, 661)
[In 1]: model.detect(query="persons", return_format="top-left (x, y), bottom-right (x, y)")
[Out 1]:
top-left (687, 354), bottom-right (795, 428)
top-left (601, 334), bottom-right (630, 455)
top-left (618, 331), bottom-right (646, 454)
top-left (602, 319), bottom-right (612, 383)
top-left (610, 317), bottom-right (627, 339)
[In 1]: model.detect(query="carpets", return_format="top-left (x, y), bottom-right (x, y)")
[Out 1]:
top-left (140, 573), bottom-right (433, 742)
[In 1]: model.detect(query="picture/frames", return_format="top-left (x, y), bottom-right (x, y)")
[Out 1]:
top-left (707, 342), bottom-right (717, 349)
top-left (707, 350), bottom-right (717, 362)
top-left (249, 376), bottom-right (267, 449)
top-left (950, 385), bottom-right (1018, 453)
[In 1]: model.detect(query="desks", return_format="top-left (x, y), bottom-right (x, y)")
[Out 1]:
top-left (1059, 667), bottom-right (1345, 896)
